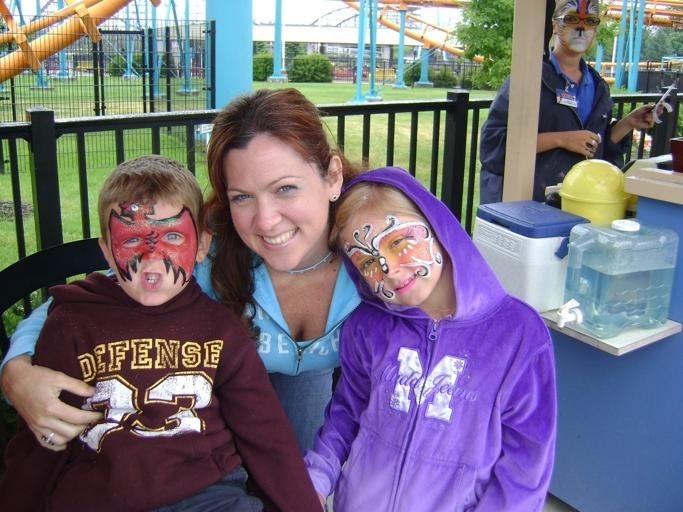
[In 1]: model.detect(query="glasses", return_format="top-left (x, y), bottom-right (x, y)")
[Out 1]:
top-left (556, 14), bottom-right (601, 26)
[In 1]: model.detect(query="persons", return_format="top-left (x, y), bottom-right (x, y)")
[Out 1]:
top-left (0, 87), bottom-right (370, 512)
top-left (303, 167), bottom-right (559, 512)
top-left (479, 0), bottom-right (663, 207)
top-left (1, 153), bottom-right (324, 512)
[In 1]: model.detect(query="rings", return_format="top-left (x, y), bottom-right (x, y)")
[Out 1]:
top-left (591, 140), bottom-right (595, 146)
top-left (46, 432), bottom-right (55, 443)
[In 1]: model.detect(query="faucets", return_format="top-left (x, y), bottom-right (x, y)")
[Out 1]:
top-left (556, 298), bottom-right (582, 330)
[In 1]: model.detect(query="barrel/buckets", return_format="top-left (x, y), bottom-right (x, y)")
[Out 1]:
top-left (564, 215), bottom-right (679, 339)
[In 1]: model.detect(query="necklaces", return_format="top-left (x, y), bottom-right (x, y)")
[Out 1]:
top-left (287, 251), bottom-right (333, 276)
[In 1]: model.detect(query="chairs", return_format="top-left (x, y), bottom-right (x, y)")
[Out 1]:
top-left (0, 238), bottom-right (109, 442)
